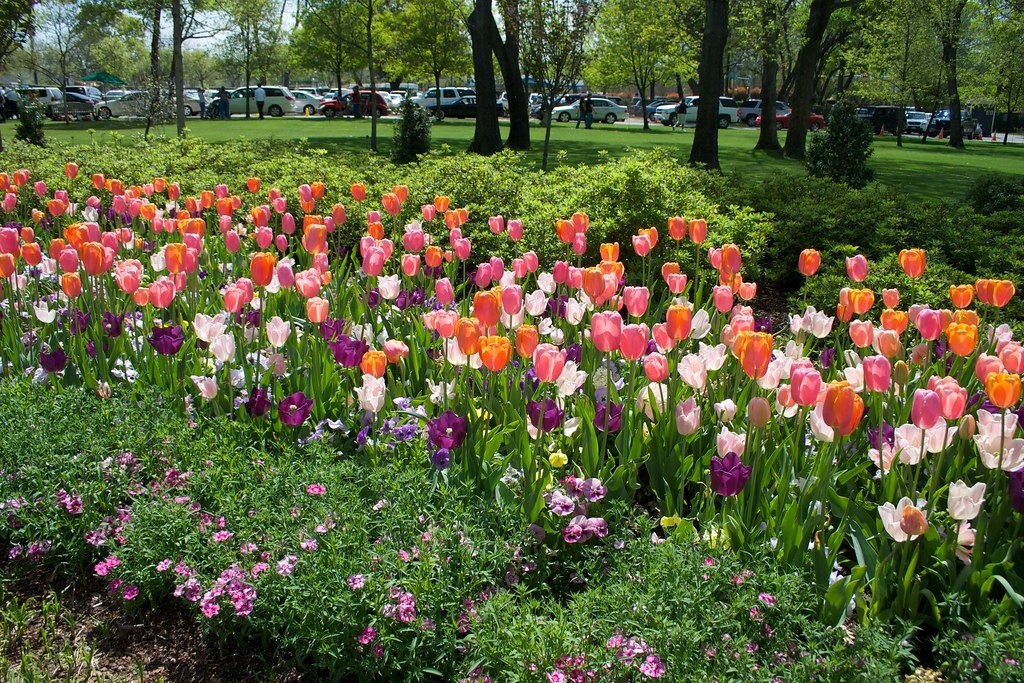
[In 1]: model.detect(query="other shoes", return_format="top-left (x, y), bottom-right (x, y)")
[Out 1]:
top-left (201, 116), bottom-right (205, 119)
top-left (681, 130), bottom-right (686, 133)
top-left (259, 116), bottom-right (264, 119)
top-left (672, 126), bottom-right (675, 133)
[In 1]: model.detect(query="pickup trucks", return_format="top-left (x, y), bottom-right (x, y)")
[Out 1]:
top-left (929, 110), bottom-right (978, 140)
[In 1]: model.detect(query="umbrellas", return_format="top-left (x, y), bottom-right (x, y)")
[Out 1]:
top-left (80, 69), bottom-right (125, 118)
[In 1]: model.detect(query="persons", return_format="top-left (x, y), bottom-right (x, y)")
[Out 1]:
top-left (575, 97), bottom-right (586, 128)
top-left (585, 93), bottom-right (598, 129)
top-left (350, 86), bottom-right (364, 119)
top-left (254, 82), bottom-right (266, 120)
top-left (0, 86), bottom-right (37, 120)
top-left (671, 99), bottom-right (688, 132)
top-left (216, 85), bottom-right (232, 120)
top-left (196, 87), bottom-right (209, 119)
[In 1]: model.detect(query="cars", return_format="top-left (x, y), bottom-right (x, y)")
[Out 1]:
top-left (1, 82), bottom-right (927, 138)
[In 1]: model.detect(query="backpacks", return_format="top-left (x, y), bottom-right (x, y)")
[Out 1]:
top-left (675, 105), bottom-right (682, 113)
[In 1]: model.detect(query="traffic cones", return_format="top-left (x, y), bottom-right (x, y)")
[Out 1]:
top-left (992, 130), bottom-right (997, 142)
top-left (880, 124), bottom-right (884, 136)
top-left (978, 129), bottom-right (983, 140)
top-left (939, 128), bottom-right (944, 139)
